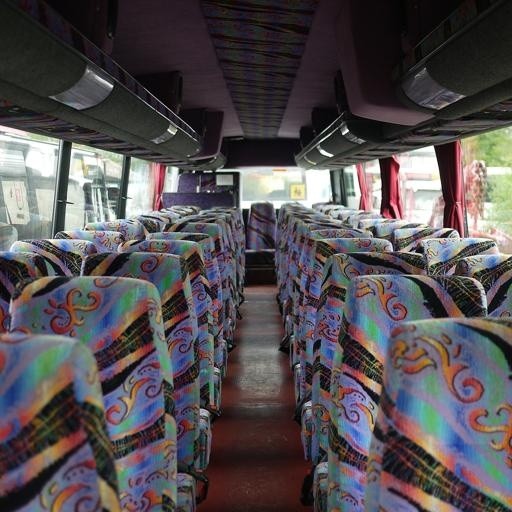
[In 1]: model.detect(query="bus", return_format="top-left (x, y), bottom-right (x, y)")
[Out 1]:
top-left (0, 129), bottom-right (152, 251)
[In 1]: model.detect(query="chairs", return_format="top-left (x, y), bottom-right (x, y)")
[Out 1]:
top-left (245, 202), bottom-right (277, 271)
top-left (0, 203), bottom-right (245, 511)
top-left (276, 202), bottom-right (512, 512)
